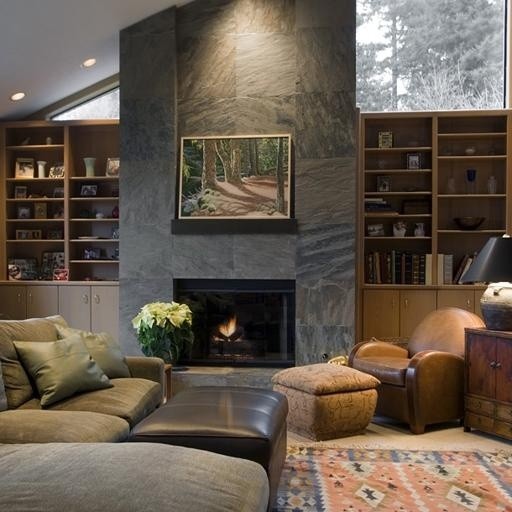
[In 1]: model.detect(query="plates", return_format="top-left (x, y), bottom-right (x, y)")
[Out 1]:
top-left (78, 235), bottom-right (99, 239)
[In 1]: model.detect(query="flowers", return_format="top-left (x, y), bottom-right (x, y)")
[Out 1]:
top-left (131, 302), bottom-right (195, 364)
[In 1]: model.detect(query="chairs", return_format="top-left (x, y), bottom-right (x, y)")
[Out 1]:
top-left (349, 306), bottom-right (486, 434)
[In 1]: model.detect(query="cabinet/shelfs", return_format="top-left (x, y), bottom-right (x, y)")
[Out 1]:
top-left (464, 328), bottom-right (512, 441)
top-left (355, 110), bottom-right (512, 342)
top-left (0, 120), bottom-right (120, 347)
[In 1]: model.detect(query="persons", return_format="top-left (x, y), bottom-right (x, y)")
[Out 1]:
top-left (393, 222), bottom-right (407, 237)
top-left (414, 225), bottom-right (425, 237)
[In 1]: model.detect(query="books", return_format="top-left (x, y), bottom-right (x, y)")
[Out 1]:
top-left (454, 254), bottom-right (469, 282)
top-left (444, 254), bottom-right (454, 284)
top-left (364, 198), bottom-right (394, 213)
top-left (438, 254), bottom-right (444, 287)
top-left (459, 257), bottom-right (472, 284)
top-left (367, 250), bottom-right (432, 286)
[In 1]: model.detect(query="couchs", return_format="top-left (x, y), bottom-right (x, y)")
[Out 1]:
top-left (0, 307), bottom-right (165, 430)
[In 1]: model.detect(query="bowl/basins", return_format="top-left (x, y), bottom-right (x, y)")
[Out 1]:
top-left (453, 216), bottom-right (487, 231)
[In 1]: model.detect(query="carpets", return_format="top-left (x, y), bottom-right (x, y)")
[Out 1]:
top-left (272, 442), bottom-right (512, 512)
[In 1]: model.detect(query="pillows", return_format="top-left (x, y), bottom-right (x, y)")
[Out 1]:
top-left (57, 323), bottom-right (133, 378)
top-left (12, 332), bottom-right (114, 407)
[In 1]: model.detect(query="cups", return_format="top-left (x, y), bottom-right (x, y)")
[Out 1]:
top-left (37, 160), bottom-right (48, 178)
top-left (83, 157), bottom-right (97, 178)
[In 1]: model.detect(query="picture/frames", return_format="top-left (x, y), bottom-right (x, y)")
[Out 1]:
top-left (178, 134), bottom-right (292, 220)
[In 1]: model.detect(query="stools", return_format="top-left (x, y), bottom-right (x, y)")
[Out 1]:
top-left (0, 441), bottom-right (269, 512)
top-left (0, 408), bottom-right (129, 445)
top-left (129, 387), bottom-right (289, 512)
top-left (271, 363), bottom-right (381, 442)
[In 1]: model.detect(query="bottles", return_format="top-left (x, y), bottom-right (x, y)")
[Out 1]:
top-left (488, 176), bottom-right (498, 195)
top-left (112, 205), bottom-right (119, 218)
top-left (465, 169), bottom-right (477, 194)
top-left (46, 137), bottom-right (52, 145)
